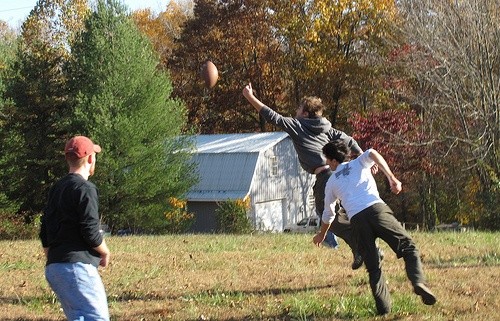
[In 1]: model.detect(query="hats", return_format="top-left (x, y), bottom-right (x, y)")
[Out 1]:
top-left (64, 135), bottom-right (102, 161)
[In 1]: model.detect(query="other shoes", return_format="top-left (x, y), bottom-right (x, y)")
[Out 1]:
top-left (351, 249), bottom-right (364, 271)
top-left (413, 282), bottom-right (437, 306)
top-left (376, 308), bottom-right (392, 316)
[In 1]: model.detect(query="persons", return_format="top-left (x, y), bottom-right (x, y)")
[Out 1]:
top-left (243, 82), bottom-right (437, 317)
top-left (39, 136), bottom-right (111, 321)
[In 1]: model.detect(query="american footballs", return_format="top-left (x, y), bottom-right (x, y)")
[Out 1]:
top-left (202, 61), bottom-right (218, 88)
